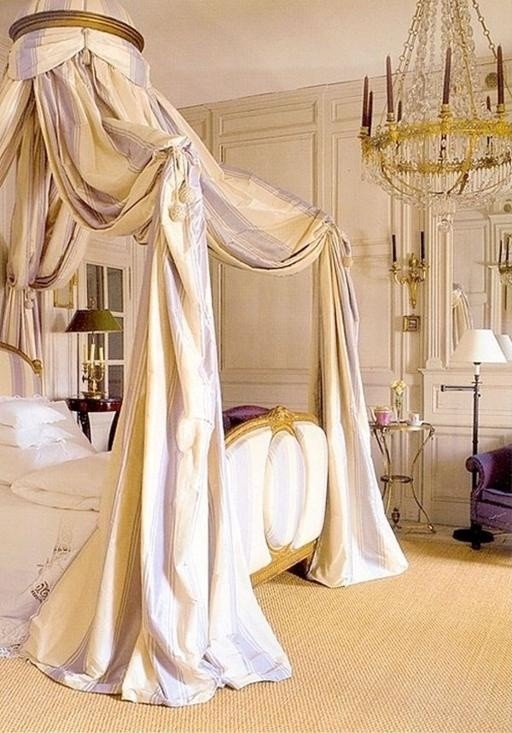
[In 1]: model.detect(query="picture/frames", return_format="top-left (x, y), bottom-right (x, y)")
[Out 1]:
top-left (53, 272), bottom-right (74, 310)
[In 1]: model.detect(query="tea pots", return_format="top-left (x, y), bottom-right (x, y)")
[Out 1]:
top-left (368, 405), bottom-right (393, 426)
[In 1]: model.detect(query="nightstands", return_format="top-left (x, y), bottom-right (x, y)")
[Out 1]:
top-left (67, 398), bottom-right (123, 451)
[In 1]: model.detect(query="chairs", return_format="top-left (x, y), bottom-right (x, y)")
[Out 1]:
top-left (465, 444), bottom-right (512, 550)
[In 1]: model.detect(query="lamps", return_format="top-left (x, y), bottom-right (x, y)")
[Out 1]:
top-left (498, 238), bottom-right (512, 286)
top-left (451, 329), bottom-right (508, 550)
top-left (65, 308), bottom-right (123, 401)
top-left (358, 1), bottom-right (512, 232)
top-left (392, 232), bottom-right (430, 309)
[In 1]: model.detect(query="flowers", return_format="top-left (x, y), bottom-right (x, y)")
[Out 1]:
top-left (390, 379), bottom-right (407, 408)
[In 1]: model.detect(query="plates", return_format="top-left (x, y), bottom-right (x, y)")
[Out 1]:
top-left (407, 422), bottom-right (422, 426)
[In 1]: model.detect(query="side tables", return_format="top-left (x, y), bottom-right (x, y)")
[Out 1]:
top-left (368, 422), bottom-right (435, 535)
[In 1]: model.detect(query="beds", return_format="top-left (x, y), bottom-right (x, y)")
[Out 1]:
top-left (1, 340), bottom-right (328, 586)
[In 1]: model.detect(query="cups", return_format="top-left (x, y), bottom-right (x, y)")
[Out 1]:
top-left (409, 413), bottom-right (419, 423)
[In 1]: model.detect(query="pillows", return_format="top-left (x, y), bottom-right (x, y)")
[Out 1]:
top-left (0, 395), bottom-right (76, 449)
